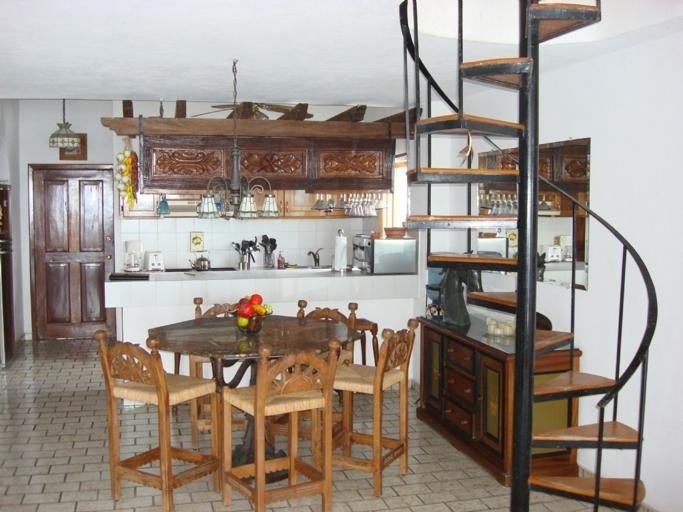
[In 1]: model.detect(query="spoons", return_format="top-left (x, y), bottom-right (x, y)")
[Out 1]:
top-left (232, 243), bottom-right (241, 252)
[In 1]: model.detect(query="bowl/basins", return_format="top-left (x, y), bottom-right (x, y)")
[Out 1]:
top-left (230, 311), bottom-right (274, 333)
top-left (384, 227), bottom-right (407, 238)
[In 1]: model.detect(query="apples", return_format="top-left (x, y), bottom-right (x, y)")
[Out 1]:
top-left (237, 300), bottom-right (253, 317)
top-left (250, 294), bottom-right (262, 305)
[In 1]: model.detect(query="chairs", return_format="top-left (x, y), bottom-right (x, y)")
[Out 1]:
top-left (188, 295), bottom-right (380, 453)
top-left (222, 338), bottom-right (339, 511)
top-left (94, 329), bottom-right (222, 511)
top-left (312, 317), bottom-right (423, 497)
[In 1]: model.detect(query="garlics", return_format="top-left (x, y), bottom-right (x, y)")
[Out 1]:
top-left (114, 149), bottom-right (130, 197)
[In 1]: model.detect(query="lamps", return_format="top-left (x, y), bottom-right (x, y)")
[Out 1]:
top-left (48, 99), bottom-right (81, 150)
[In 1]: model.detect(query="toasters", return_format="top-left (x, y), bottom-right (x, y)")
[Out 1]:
top-left (145, 251), bottom-right (164, 271)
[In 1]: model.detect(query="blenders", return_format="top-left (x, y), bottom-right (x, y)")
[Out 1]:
top-left (123, 240), bottom-right (144, 272)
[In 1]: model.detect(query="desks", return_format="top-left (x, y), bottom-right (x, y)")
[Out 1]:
top-left (148, 314), bottom-right (365, 486)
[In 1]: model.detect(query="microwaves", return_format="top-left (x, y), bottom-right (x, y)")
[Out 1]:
top-left (352, 234), bottom-right (417, 274)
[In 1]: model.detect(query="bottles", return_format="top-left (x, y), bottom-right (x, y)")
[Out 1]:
top-left (479, 183), bottom-right (518, 215)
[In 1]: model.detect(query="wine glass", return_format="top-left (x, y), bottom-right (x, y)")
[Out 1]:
top-left (339, 193), bottom-right (380, 216)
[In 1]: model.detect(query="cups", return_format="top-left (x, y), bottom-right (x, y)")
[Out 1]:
top-left (371, 232), bottom-right (380, 239)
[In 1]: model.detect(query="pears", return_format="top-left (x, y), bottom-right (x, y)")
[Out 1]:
top-left (237, 316), bottom-right (248, 327)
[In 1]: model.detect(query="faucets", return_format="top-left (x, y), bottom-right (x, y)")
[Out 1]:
top-left (307, 248), bottom-right (323, 267)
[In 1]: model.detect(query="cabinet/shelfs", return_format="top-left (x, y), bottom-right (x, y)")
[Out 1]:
top-left (140, 133), bottom-right (397, 196)
top-left (125, 134), bottom-right (161, 218)
top-left (195, 59), bottom-right (281, 222)
top-left (162, 193), bottom-right (233, 218)
top-left (256, 189), bottom-right (325, 218)
top-left (326, 190), bottom-right (377, 217)
top-left (415, 311), bottom-right (583, 489)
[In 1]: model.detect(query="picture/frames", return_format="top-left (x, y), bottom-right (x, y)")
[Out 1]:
top-left (60, 133), bottom-right (87, 160)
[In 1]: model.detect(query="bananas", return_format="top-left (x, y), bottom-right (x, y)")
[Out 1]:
top-left (252, 304), bottom-right (266, 316)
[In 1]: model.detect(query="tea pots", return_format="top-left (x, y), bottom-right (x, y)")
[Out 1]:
top-left (188, 254), bottom-right (210, 271)
